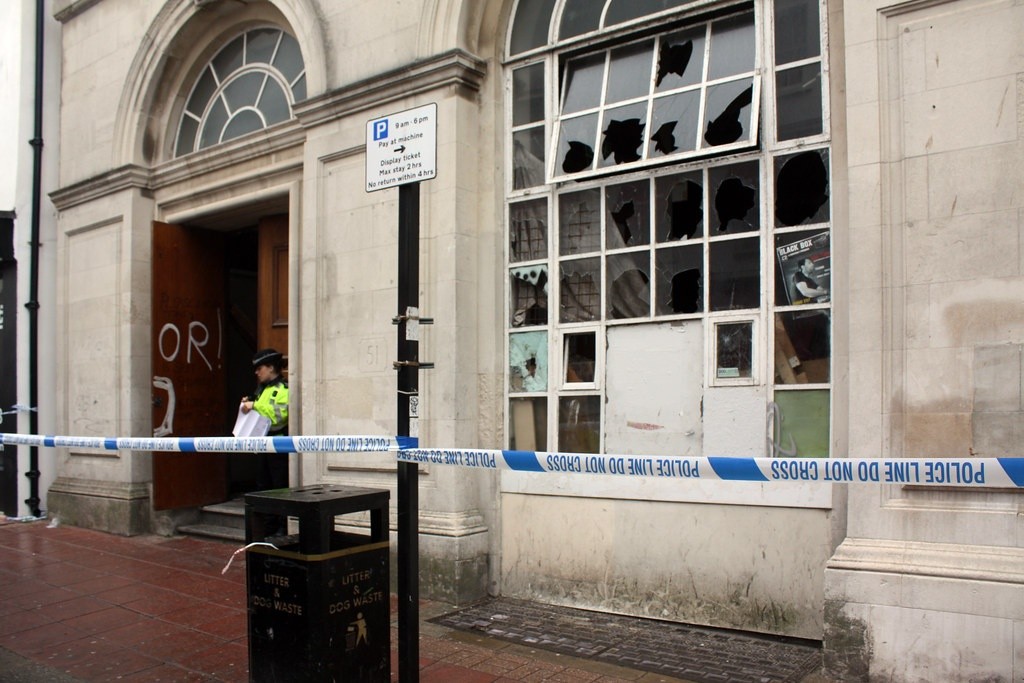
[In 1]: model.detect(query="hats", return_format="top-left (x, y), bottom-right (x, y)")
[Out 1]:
top-left (252, 348), bottom-right (283, 369)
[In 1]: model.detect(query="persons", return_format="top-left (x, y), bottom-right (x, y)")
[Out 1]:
top-left (241, 348), bottom-right (288, 491)
top-left (792, 257), bottom-right (830, 306)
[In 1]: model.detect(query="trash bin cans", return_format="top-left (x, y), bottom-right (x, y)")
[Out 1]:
top-left (239, 481), bottom-right (391, 682)
